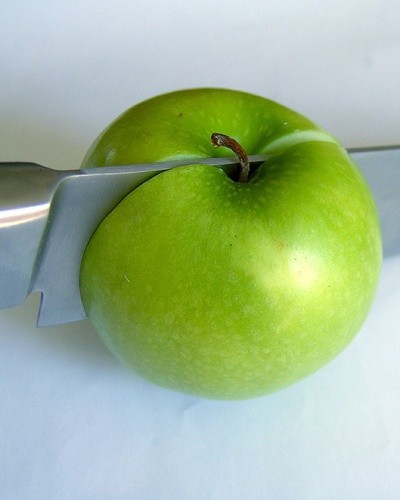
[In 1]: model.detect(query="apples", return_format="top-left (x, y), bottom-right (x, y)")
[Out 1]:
top-left (80, 87), bottom-right (384, 395)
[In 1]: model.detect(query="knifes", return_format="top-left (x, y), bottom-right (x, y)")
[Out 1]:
top-left (1, 144), bottom-right (400, 328)
